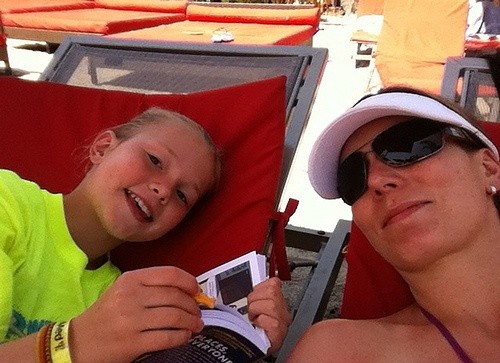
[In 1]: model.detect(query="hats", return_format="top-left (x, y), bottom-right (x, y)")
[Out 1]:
top-left (308, 93), bottom-right (499, 200)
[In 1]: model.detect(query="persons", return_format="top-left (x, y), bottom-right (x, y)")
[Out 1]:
top-left (0, 104), bottom-right (289, 362)
top-left (280, 87), bottom-right (498, 361)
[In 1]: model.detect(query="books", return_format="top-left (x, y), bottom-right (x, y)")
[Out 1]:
top-left (130, 252), bottom-right (273, 362)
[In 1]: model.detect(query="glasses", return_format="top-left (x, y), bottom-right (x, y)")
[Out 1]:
top-left (337, 119), bottom-right (446, 206)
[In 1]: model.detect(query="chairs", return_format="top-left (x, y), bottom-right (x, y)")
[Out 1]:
top-left (0, 0), bottom-right (500, 363)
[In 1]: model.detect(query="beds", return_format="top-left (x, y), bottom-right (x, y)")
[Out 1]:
top-left (0, 0), bottom-right (190, 85)
top-left (87, 2), bottom-right (323, 86)
top-left (0, 0), bottom-right (95, 76)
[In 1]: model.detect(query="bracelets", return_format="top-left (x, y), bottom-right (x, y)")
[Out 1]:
top-left (37, 316), bottom-right (75, 363)
